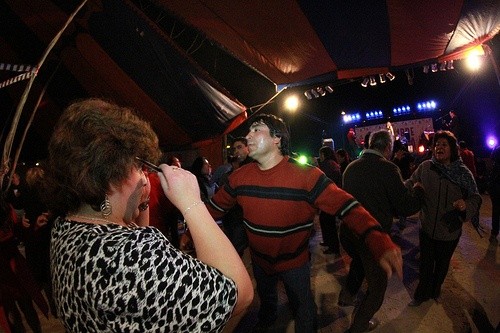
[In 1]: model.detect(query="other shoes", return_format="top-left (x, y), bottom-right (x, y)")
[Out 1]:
top-left (408, 297), bottom-right (423, 307)
top-left (338, 297), bottom-right (359, 304)
top-left (352, 319), bottom-right (378, 332)
top-left (323, 248), bottom-right (337, 254)
top-left (319, 242), bottom-right (328, 246)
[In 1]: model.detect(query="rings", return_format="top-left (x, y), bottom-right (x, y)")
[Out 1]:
top-left (460, 205), bottom-right (462, 208)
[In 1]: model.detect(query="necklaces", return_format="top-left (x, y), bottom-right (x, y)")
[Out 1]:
top-left (65, 213), bottom-right (119, 225)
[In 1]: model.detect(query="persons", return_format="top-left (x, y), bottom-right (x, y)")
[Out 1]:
top-left (0, 100), bottom-right (500, 333)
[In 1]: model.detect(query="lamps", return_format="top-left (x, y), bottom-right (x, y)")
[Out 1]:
top-left (439, 61), bottom-right (447, 71)
top-left (303, 90), bottom-right (313, 101)
top-left (430, 63), bottom-right (438, 73)
top-left (325, 86), bottom-right (335, 93)
top-left (386, 72), bottom-right (396, 82)
top-left (379, 73), bottom-right (386, 83)
top-left (447, 60), bottom-right (455, 70)
top-left (423, 64), bottom-right (429, 73)
top-left (310, 89), bottom-right (319, 98)
top-left (361, 78), bottom-right (370, 87)
top-left (369, 76), bottom-right (377, 86)
top-left (317, 87), bottom-right (326, 97)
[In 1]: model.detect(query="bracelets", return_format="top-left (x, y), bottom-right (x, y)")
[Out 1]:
top-left (183, 201), bottom-right (205, 232)
top-left (138, 198), bottom-right (150, 212)
